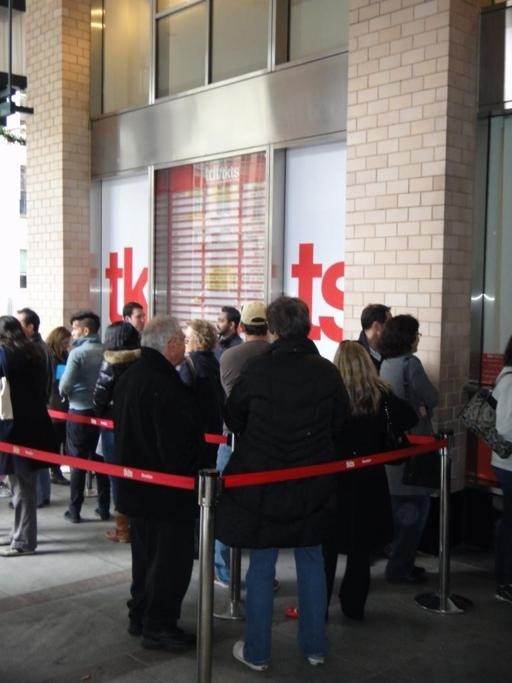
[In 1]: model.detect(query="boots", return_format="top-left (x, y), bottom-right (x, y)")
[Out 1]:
top-left (105, 513), bottom-right (129, 543)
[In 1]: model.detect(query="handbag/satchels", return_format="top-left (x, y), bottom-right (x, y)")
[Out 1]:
top-left (457, 389), bottom-right (512, 458)
top-left (385, 423), bottom-right (411, 465)
top-left (0, 376), bottom-right (14, 420)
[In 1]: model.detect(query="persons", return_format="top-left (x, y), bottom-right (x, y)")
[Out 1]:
top-left (214, 296), bottom-right (353, 671)
top-left (284, 340), bottom-right (419, 624)
top-left (112, 317), bottom-right (205, 653)
top-left (1, 301), bottom-right (442, 594)
top-left (490, 335), bottom-right (512, 605)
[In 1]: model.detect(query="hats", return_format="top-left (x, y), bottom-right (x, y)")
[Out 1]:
top-left (241, 302), bottom-right (268, 325)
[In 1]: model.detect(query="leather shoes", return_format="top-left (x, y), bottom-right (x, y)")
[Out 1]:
top-left (386, 562), bottom-right (425, 583)
top-left (50, 470), bottom-right (71, 485)
top-left (285, 607), bottom-right (298, 617)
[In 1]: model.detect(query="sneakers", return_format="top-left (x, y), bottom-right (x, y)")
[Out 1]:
top-left (233, 640), bottom-right (268, 671)
top-left (128, 617), bottom-right (195, 650)
top-left (307, 656), bottom-right (325, 665)
top-left (95, 509), bottom-right (109, 521)
top-left (496, 584), bottom-right (512, 602)
top-left (0, 536), bottom-right (35, 556)
top-left (65, 510), bottom-right (80, 523)
